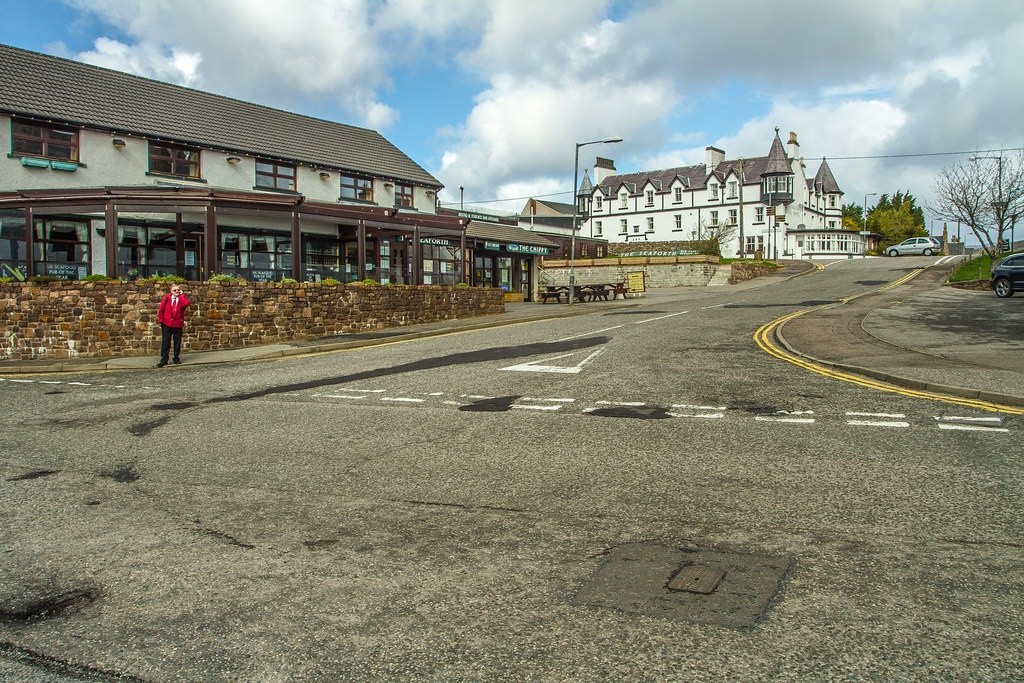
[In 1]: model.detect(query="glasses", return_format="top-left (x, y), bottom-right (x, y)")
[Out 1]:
top-left (172, 289), bottom-right (181, 292)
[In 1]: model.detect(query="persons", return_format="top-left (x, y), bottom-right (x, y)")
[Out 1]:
top-left (157, 284), bottom-right (190, 366)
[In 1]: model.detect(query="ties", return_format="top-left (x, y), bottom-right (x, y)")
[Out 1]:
top-left (172, 297), bottom-right (176, 319)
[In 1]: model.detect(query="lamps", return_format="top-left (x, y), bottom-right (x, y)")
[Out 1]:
top-left (113, 139), bottom-right (126, 147)
top-left (319, 172), bottom-right (330, 179)
top-left (227, 157), bottom-right (241, 163)
top-left (384, 183), bottom-right (393, 189)
top-left (426, 191), bottom-right (435, 196)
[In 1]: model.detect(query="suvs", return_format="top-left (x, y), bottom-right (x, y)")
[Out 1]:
top-left (989, 252), bottom-right (1024, 298)
top-left (882, 237), bottom-right (942, 256)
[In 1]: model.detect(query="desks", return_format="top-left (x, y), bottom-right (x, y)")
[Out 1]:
top-left (538, 282), bottom-right (625, 289)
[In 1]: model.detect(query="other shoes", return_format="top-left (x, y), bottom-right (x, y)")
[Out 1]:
top-left (174, 360), bottom-right (181, 364)
top-left (157, 361), bottom-right (167, 367)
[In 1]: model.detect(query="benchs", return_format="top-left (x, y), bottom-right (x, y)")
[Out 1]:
top-left (536, 287), bottom-right (632, 304)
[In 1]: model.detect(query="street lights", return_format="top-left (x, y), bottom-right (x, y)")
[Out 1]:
top-left (457, 185), bottom-right (465, 211)
top-left (964, 232), bottom-right (973, 254)
top-left (863, 193), bottom-right (877, 258)
top-left (587, 196), bottom-right (594, 239)
top-left (931, 217), bottom-right (942, 236)
top-left (969, 156), bottom-right (1003, 255)
top-left (568, 137), bottom-right (625, 307)
